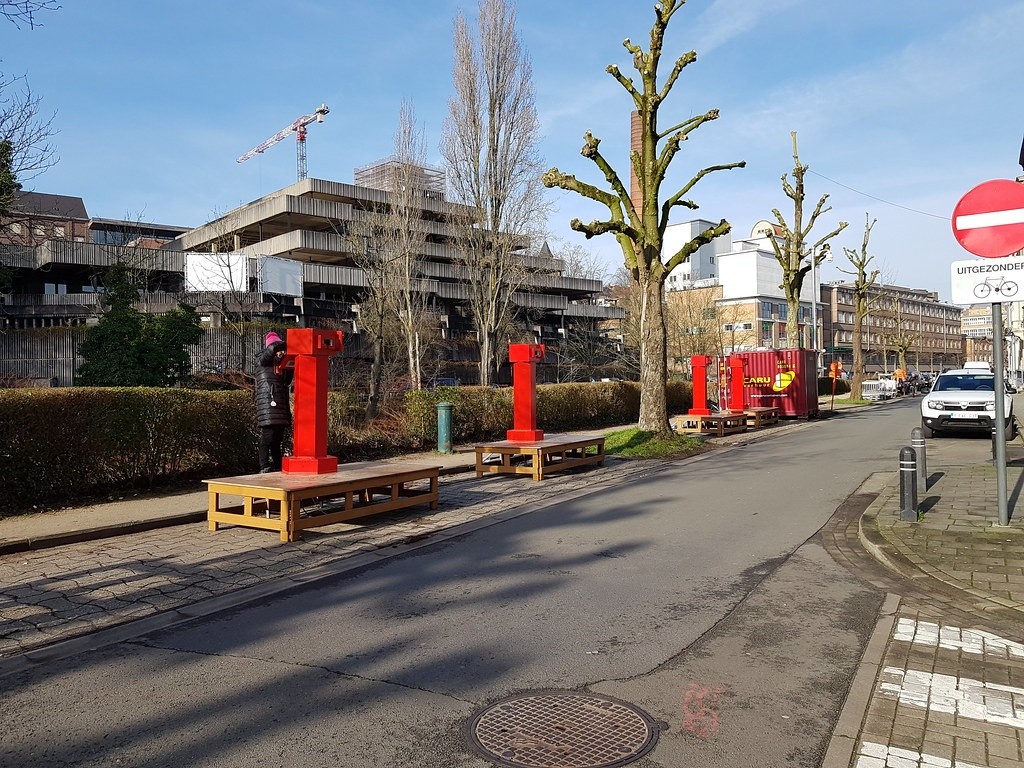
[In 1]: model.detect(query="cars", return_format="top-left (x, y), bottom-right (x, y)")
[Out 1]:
top-left (919, 372), bottom-right (1014, 441)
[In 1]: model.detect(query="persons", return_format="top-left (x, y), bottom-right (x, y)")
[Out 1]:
top-left (254, 332), bottom-right (291, 473)
top-left (880, 366), bottom-right (918, 398)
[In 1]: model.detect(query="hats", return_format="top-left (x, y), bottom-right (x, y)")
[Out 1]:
top-left (264, 332), bottom-right (282, 349)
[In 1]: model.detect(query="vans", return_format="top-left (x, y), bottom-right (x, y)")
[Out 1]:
top-left (964, 361), bottom-right (991, 373)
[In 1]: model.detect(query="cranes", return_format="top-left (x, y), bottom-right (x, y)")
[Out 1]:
top-left (236, 103), bottom-right (331, 181)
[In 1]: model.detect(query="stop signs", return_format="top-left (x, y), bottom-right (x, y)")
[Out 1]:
top-left (951, 179), bottom-right (1024, 256)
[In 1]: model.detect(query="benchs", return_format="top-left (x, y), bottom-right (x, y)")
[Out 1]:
top-left (473, 434), bottom-right (607, 480)
top-left (199, 461), bottom-right (443, 542)
top-left (860, 380), bottom-right (896, 400)
top-left (677, 409), bottom-right (780, 438)
